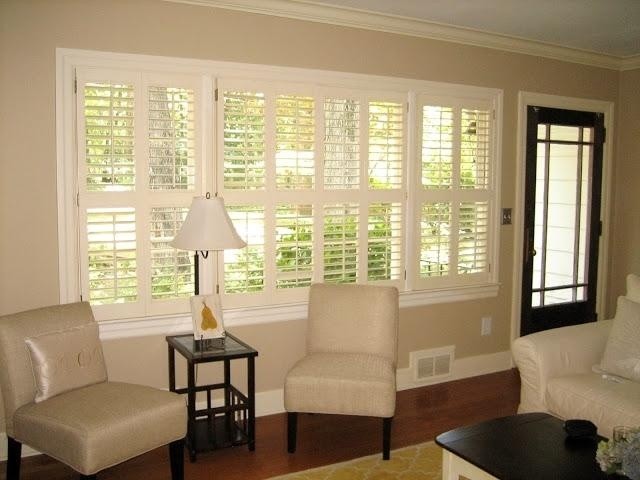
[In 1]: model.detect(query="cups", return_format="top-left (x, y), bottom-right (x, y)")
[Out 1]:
top-left (613, 425), bottom-right (632, 446)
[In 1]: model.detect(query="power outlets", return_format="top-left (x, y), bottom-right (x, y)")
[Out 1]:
top-left (481, 318), bottom-right (492, 336)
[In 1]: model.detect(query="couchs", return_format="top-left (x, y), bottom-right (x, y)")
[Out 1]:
top-left (512, 274), bottom-right (640, 439)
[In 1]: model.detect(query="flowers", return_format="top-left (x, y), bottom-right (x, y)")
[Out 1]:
top-left (595, 426), bottom-right (640, 480)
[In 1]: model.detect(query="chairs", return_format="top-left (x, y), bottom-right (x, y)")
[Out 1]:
top-left (284, 284), bottom-right (399, 460)
top-left (1, 301), bottom-right (188, 480)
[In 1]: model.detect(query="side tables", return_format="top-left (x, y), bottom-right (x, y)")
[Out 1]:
top-left (166, 330), bottom-right (258, 462)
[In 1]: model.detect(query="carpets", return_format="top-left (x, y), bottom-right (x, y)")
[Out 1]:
top-left (265, 440), bottom-right (444, 479)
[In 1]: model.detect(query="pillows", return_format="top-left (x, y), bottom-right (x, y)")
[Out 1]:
top-left (601, 296), bottom-right (640, 382)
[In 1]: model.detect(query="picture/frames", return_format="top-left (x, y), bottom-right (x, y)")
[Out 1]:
top-left (189, 295), bottom-right (226, 340)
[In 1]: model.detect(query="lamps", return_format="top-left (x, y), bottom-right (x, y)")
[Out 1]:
top-left (168, 195), bottom-right (248, 295)
